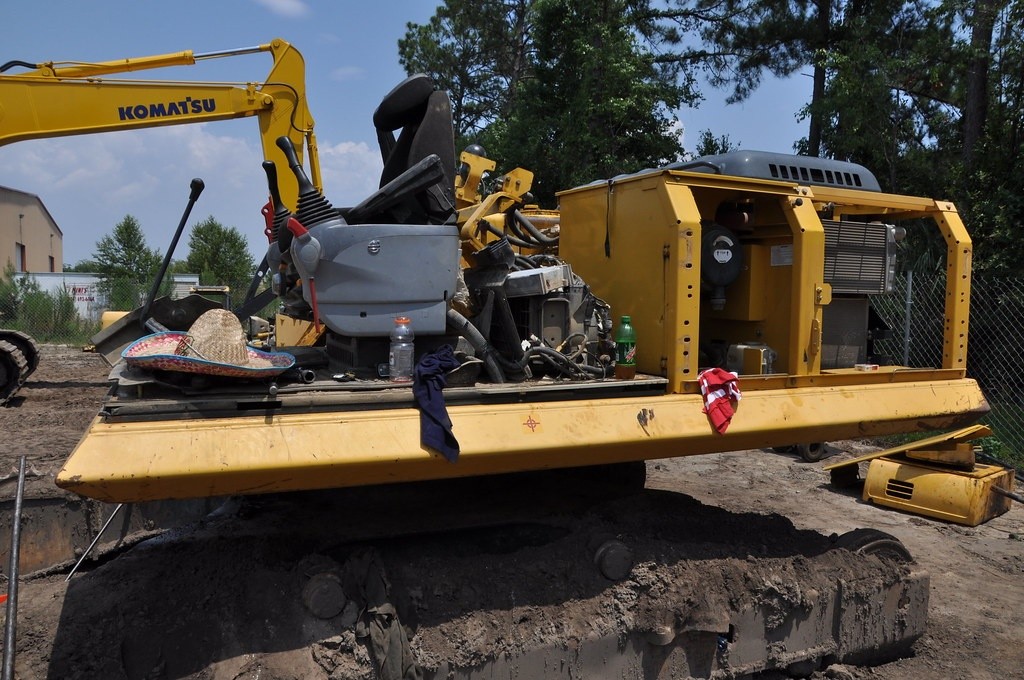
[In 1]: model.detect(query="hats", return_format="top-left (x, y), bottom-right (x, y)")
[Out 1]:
top-left (120, 308), bottom-right (296, 378)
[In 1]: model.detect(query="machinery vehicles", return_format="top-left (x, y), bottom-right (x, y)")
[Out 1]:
top-left (0, 38), bottom-right (327, 404)
top-left (52, 135), bottom-right (992, 680)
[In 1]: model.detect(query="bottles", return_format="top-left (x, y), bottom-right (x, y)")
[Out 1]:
top-left (616, 315), bottom-right (637, 379)
top-left (389, 315), bottom-right (414, 381)
